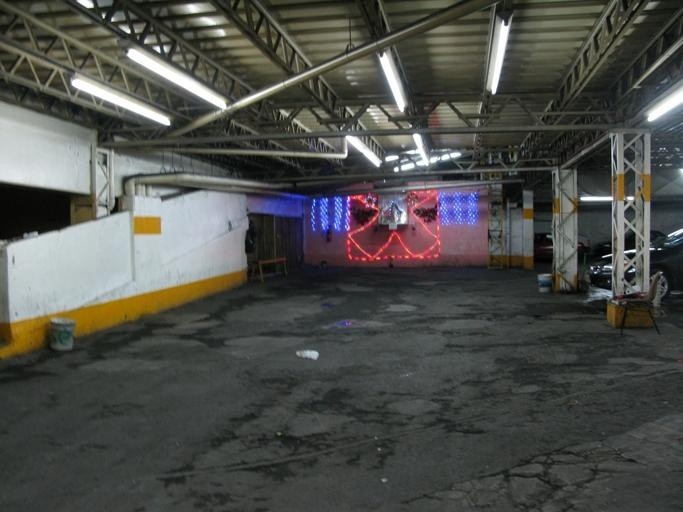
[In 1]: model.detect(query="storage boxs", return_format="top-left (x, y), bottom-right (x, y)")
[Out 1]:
top-left (606, 297), bottom-right (654, 329)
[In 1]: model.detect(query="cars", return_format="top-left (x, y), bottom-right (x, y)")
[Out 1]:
top-left (588, 229), bottom-right (683, 301)
top-left (535, 230), bottom-right (589, 258)
top-left (595, 229), bottom-right (664, 258)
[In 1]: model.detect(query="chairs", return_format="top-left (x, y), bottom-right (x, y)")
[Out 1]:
top-left (618, 271), bottom-right (662, 336)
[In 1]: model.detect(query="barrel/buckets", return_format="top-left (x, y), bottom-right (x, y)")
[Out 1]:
top-left (536, 274), bottom-right (553, 292)
top-left (49, 316), bottom-right (76, 350)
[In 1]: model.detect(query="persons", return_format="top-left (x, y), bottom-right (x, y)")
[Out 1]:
top-left (246, 221), bottom-right (257, 282)
